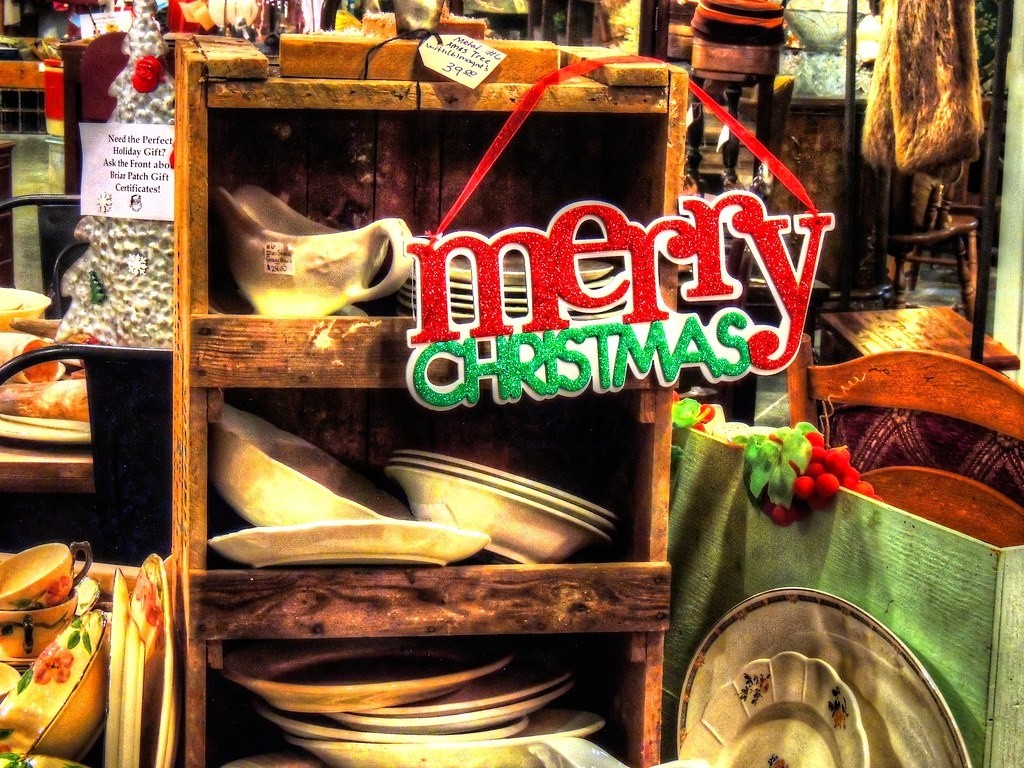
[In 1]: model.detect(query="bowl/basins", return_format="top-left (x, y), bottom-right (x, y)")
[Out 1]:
top-left (783, 9), bottom-right (867, 49)
top-left (0, 608), bottom-right (111, 762)
top-left (385, 448), bottom-right (618, 564)
top-left (0, 286), bottom-right (53, 330)
top-left (210, 404), bottom-right (412, 527)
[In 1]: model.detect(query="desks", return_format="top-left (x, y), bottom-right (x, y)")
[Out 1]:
top-left (58, 37), bottom-right (94, 195)
top-left (824, 304), bottom-right (1020, 376)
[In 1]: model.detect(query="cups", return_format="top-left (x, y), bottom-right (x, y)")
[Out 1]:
top-left (0, 541), bottom-right (91, 611)
top-left (0, 590), bottom-right (78, 657)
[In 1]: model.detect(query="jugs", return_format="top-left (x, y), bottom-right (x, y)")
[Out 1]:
top-left (214, 183), bottom-right (413, 319)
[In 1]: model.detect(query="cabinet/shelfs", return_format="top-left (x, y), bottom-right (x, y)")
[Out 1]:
top-left (169, 42), bottom-right (689, 768)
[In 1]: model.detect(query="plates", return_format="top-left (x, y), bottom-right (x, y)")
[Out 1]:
top-left (209, 522), bottom-right (492, 570)
top-left (104, 553), bottom-right (179, 768)
top-left (218, 637), bottom-right (607, 768)
top-left (679, 650), bottom-right (872, 768)
top-left (677, 586), bottom-right (973, 768)
top-left (0, 333), bottom-right (91, 446)
top-left (395, 254), bottom-right (625, 320)
top-left (73, 574), bottom-right (101, 615)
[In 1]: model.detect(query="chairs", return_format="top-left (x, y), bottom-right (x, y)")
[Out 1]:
top-left (785, 333), bottom-right (1024, 546)
top-left (0, 195), bottom-right (171, 566)
top-left (889, 214), bottom-right (980, 321)
